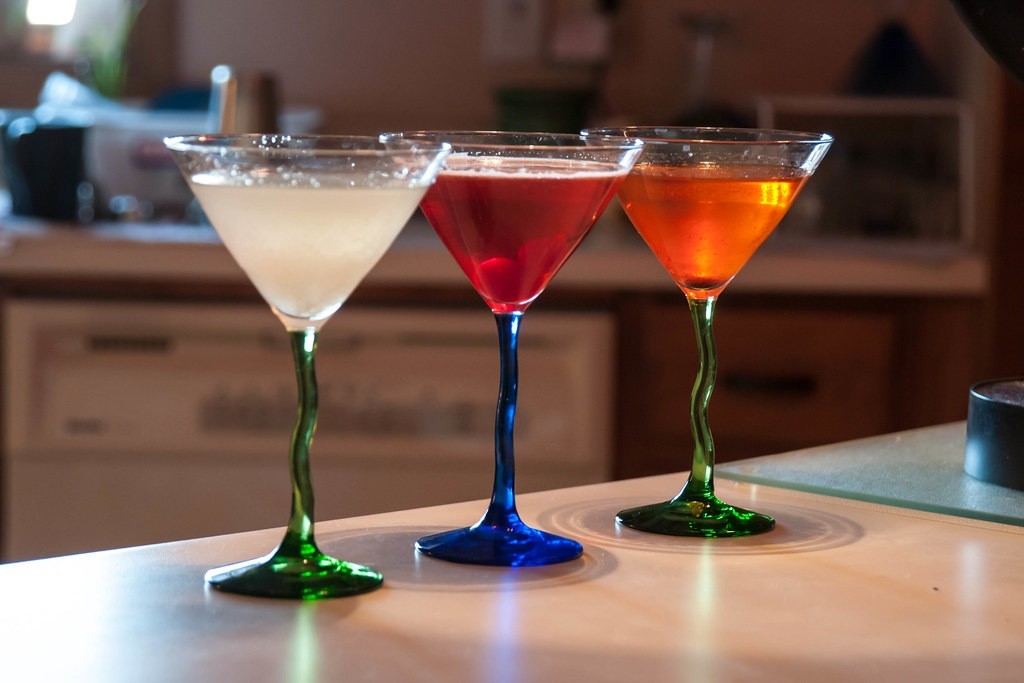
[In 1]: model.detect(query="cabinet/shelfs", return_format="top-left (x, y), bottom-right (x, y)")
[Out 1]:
top-left (631, 303), bottom-right (995, 472)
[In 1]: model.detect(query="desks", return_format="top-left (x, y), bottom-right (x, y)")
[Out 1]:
top-left (0, 473), bottom-right (1023, 683)
top-left (716, 416), bottom-right (1024, 526)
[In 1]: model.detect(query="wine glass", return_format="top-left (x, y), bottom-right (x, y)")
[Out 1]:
top-left (165, 134), bottom-right (455, 598)
top-left (581, 125), bottom-right (835, 539)
top-left (379, 129), bottom-right (645, 567)
